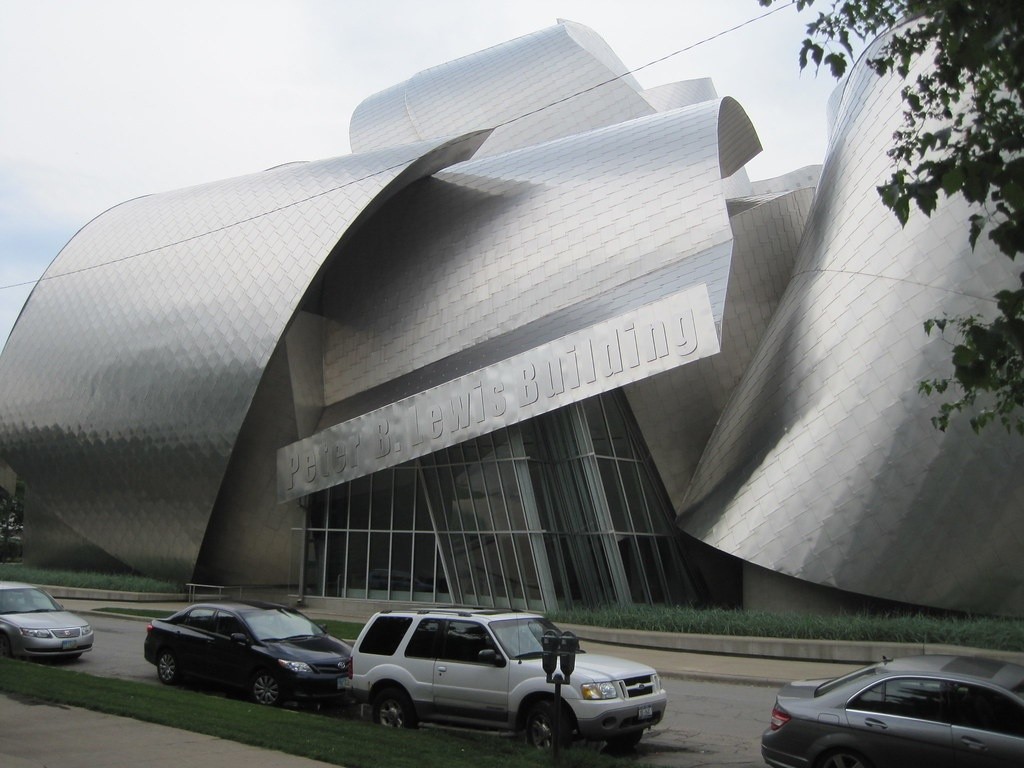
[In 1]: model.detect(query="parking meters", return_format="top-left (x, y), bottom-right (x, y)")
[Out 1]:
top-left (540, 630), bottom-right (579, 768)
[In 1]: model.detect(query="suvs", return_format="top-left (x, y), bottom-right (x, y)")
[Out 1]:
top-left (349, 608), bottom-right (667, 750)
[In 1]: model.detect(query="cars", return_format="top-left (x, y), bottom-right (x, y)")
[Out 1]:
top-left (143, 600), bottom-right (352, 706)
top-left (761, 654), bottom-right (1024, 768)
top-left (0, 581), bottom-right (94, 659)
